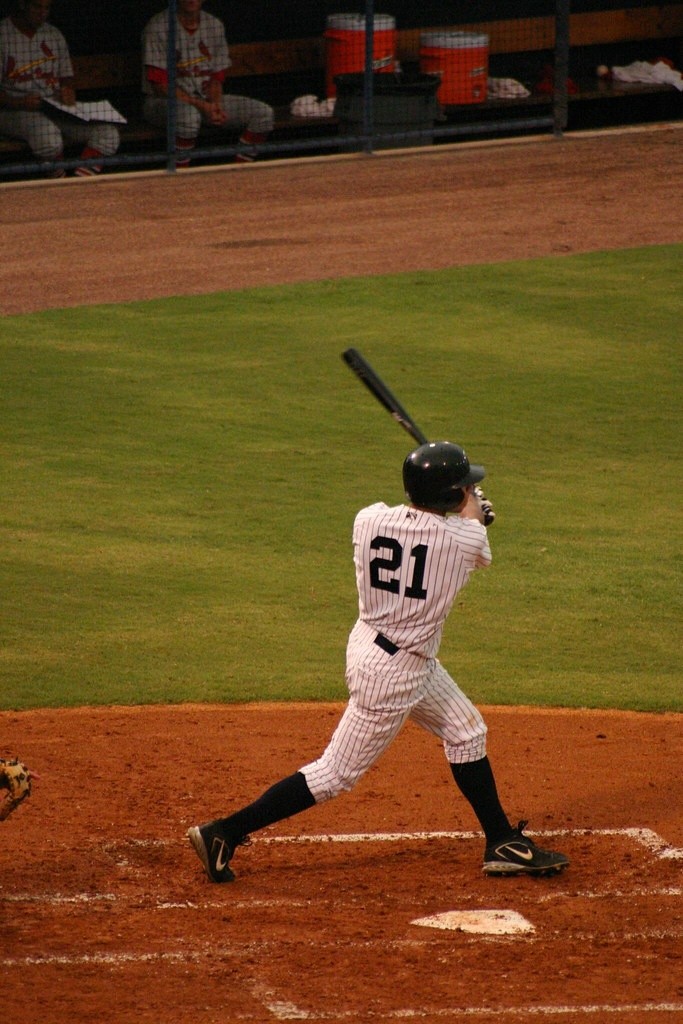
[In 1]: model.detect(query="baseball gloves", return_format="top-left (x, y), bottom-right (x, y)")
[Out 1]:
top-left (0, 745), bottom-right (33, 822)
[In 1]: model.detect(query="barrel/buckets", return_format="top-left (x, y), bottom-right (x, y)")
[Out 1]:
top-left (419, 31), bottom-right (490, 103)
top-left (325, 14), bottom-right (396, 100)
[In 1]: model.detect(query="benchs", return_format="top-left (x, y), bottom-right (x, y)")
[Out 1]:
top-left (0, 5), bottom-right (683, 155)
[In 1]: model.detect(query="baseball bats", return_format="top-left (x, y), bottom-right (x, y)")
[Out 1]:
top-left (339, 344), bottom-right (495, 525)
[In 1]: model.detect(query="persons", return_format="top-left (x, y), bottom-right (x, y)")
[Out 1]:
top-left (142, 0), bottom-right (275, 168)
top-left (186, 441), bottom-right (572, 884)
top-left (0, 0), bottom-right (120, 178)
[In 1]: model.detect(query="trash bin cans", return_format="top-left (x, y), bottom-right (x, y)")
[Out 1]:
top-left (332, 72), bottom-right (440, 155)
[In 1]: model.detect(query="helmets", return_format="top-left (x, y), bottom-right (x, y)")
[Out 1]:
top-left (402, 441), bottom-right (485, 511)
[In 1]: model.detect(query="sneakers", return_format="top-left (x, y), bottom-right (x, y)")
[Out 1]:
top-left (483, 819), bottom-right (570, 879)
top-left (188, 821), bottom-right (235, 883)
